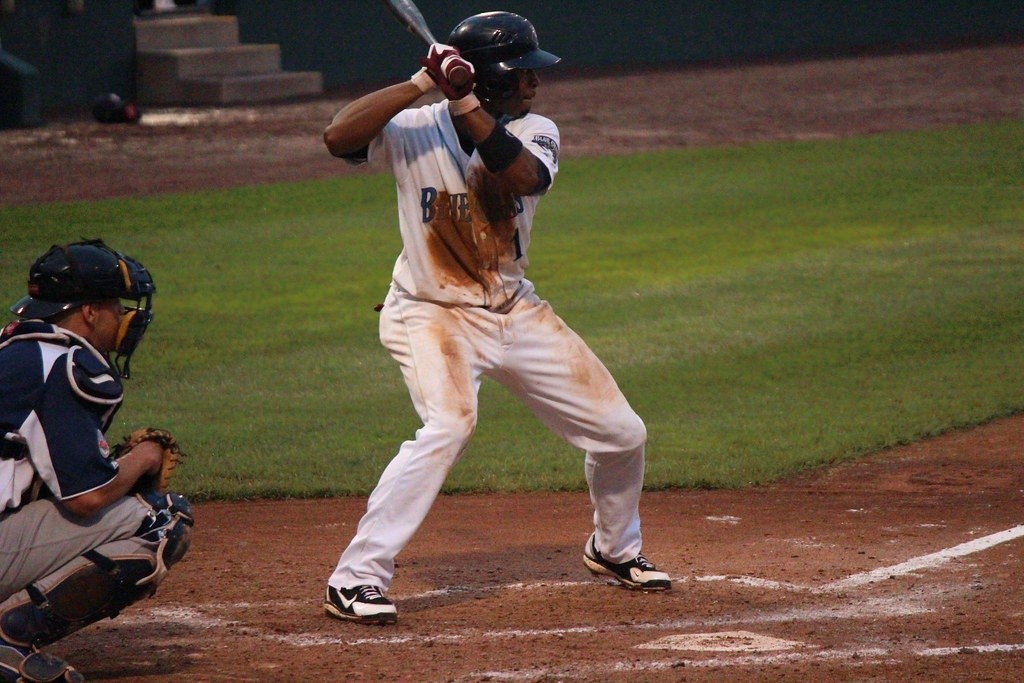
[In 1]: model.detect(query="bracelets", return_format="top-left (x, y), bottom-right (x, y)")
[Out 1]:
top-left (410, 67), bottom-right (435, 95)
top-left (475, 121), bottom-right (522, 173)
top-left (447, 91), bottom-right (482, 118)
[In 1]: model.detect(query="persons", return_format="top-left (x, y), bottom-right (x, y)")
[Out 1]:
top-left (0, 236), bottom-right (195, 683)
top-left (322, 12), bottom-right (673, 623)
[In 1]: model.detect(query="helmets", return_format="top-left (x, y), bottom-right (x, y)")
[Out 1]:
top-left (8, 237), bottom-right (157, 380)
top-left (447, 11), bottom-right (562, 102)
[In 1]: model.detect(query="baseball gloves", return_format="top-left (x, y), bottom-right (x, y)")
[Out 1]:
top-left (111, 426), bottom-right (182, 496)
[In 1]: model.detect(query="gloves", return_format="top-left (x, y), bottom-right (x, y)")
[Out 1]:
top-left (411, 43), bottom-right (459, 94)
top-left (418, 50), bottom-right (480, 117)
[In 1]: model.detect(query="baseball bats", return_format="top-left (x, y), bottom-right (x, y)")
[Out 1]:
top-left (383, 1), bottom-right (470, 90)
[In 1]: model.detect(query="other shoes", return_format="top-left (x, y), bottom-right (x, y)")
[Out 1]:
top-left (93, 92), bottom-right (143, 126)
top-left (0, 630), bottom-right (84, 683)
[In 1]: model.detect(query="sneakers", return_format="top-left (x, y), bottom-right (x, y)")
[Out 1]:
top-left (583, 531), bottom-right (672, 594)
top-left (323, 584), bottom-right (398, 624)
